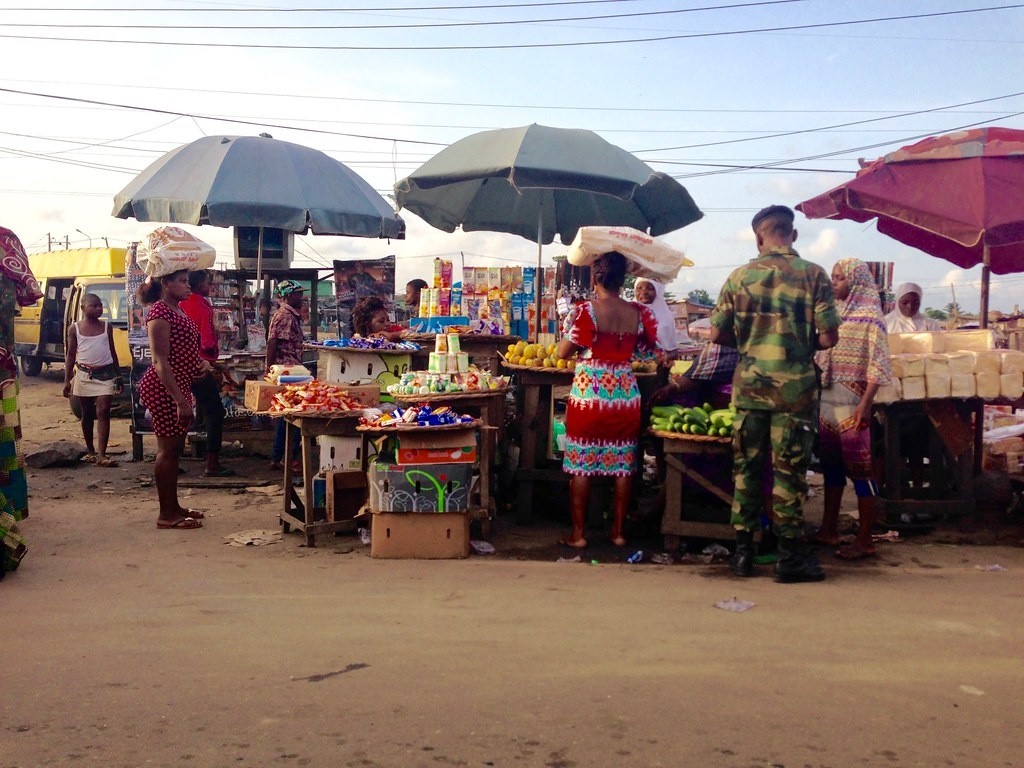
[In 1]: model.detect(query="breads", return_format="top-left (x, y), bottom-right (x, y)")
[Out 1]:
top-left (872, 329), bottom-right (1023, 403)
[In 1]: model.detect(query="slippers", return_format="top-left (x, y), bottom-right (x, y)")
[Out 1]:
top-left (609, 535), bottom-right (628, 548)
top-left (156, 516), bottom-right (203, 529)
top-left (555, 539), bottom-right (587, 551)
top-left (202, 468), bottom-right (237, 477)
top-left (180, 508), bottom-right (203, 520)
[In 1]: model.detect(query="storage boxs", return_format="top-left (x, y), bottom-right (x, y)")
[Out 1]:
top-left (371, 513), bottom-right (471, 559)
top-left (547, 383), bottom-right (569, 459)
top-left (396, 429), bottom-right (475, 464)
top-left (337, 384), bottom-right (377, 405)
top-left (370, 464), bottom-right (473, 513)
top-left (243, 379), bottom-right (287, 412)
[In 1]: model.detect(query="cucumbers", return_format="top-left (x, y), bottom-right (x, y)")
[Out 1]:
top-left (651, 402), bottom-right (738, 437)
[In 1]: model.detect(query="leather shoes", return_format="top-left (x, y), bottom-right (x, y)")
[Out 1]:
top-left (773, 558), bottom-right (826, 582)
top-left (731, 553), bottom-right (756, 576)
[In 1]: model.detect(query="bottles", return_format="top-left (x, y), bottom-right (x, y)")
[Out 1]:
top-left (402, 333), bottom-right (468, 393)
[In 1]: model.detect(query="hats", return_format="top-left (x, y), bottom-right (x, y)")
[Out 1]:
top-left (274, 279), bottom-right (310, 297)
top-left (752, 205), bottom-right (795, 231)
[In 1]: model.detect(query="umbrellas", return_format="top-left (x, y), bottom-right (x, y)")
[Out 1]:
top-left (110, 134), bottom-right (406, 324)
top-left (394, 123), bottom-right (706, 340)
top-left (792, 127), bottom-right (1024, 476)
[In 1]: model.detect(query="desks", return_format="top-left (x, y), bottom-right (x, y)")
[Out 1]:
top-left (661, 436), bottom-right (765, 548)
top-left (283, 413), bottom-right (499, 546)
top-left (419, 343), bottom-right (516, 472)
top-left (881, 400), bottom-right (987, 526)
top-left (522, 373), bottom-right (671, 521)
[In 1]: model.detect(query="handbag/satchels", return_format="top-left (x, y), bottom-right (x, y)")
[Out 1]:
top-left (76, 362), bottom-right (115, 381)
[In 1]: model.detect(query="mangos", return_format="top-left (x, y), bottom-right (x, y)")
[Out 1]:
top-left (504, 340), bottom-right (652, 372)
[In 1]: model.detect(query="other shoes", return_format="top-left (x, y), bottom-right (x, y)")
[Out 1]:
top-left (85, 455), bottom-right (97, 463)
top-left (808, 531), bottom-right (840, 547)
top-left (834, 540), bottom-right (879, 560)
top-left (94, 459), bottom-right (118, 467)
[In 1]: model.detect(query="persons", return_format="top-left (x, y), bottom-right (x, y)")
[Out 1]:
top-left (807, 258), bottom-right (893, 560)
top-left (351, 298), bottom-right (408, 341)
top-left (62, 294), bottom-right (123, 466)
top-left (266, 280), bottom-right (309, 473)
top-left (880, 281), bottom-right (943, 492)
top-left (319, 307), bottom-right (338, 336)
top-left (349, 261), bottom-right (392, 298)
top-left (630, 276), bottom-right (690, 350)
top-left (710, 206), bottom-right (840, 581)
top-left (556, 251), bottom-right (655, 550)
top-left (137, 269), bottom-right (235, 528)
top-left (0, 227), bottom-right (48, 580)
top-left (405, 279), bottom-right (429, 316)
